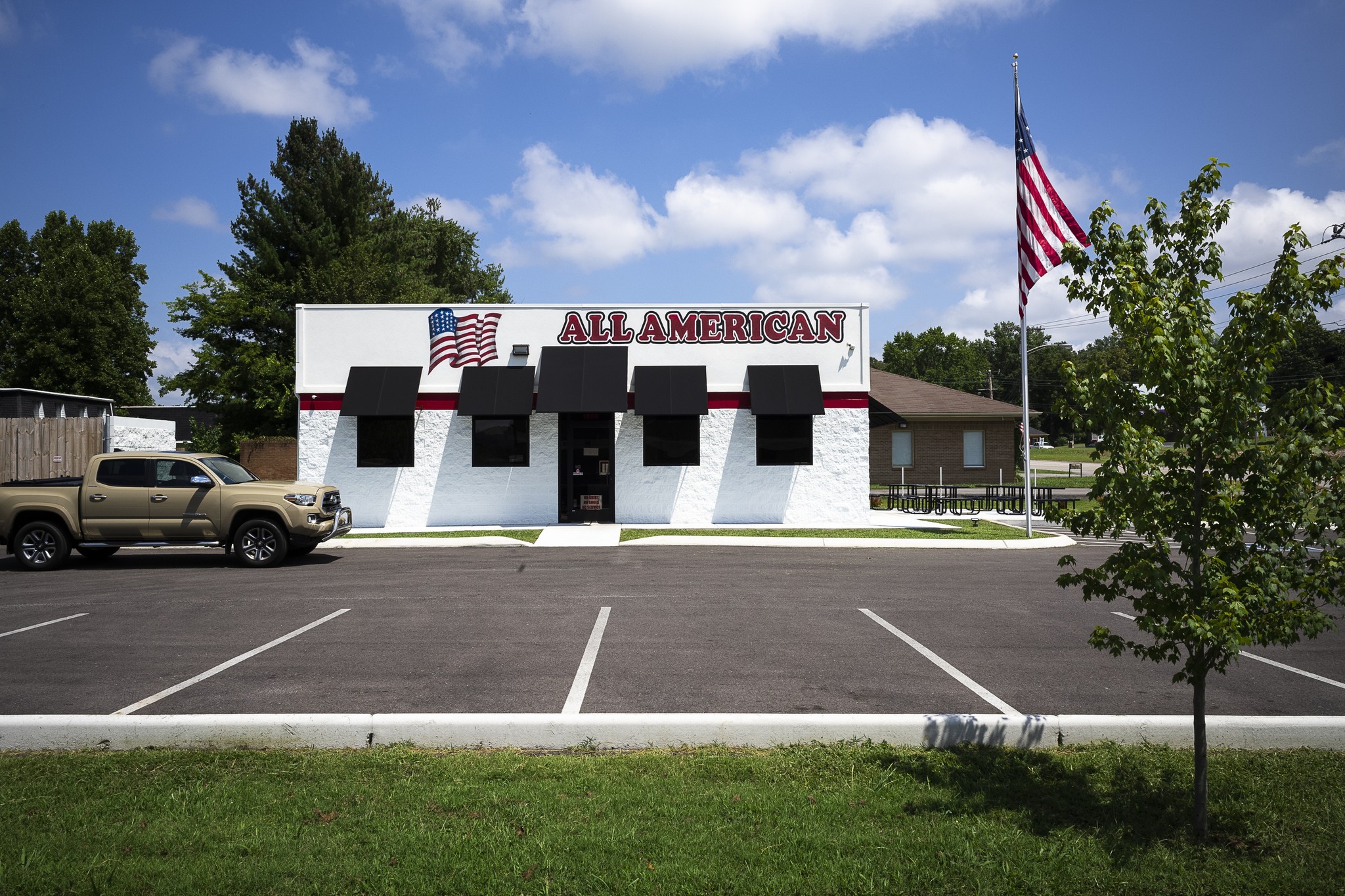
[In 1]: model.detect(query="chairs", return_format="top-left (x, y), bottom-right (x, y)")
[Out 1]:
top-left (165, 462), bottom-right (200, 485)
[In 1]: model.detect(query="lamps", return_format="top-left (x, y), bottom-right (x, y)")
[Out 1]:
top-left (899, 422), bottom-right (908, 429)
top-left (971, 518), bottom-right (979, 522)
top-left (512, 344), bottom-right (531, 356)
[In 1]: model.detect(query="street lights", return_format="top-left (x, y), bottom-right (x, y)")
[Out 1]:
top-left (1021, 343), bottom-right (1073, 537)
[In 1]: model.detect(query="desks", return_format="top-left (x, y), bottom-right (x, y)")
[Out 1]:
top-left (976, 485), bottom-right (1066, 516)
top-left (887, 484), bottom-right (970, 515)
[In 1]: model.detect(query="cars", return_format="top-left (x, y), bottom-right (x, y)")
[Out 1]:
top-left (1085, 439), bottom-right (1101, 447)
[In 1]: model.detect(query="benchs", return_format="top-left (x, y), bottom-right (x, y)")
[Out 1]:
top-left (870, 493), bottom-right (1080, 515)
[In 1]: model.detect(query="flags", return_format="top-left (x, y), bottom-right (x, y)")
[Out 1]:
top-left (428, 308), bottom-right (502, 376)
top-left (1012, 85), bottom-right (1093, 318)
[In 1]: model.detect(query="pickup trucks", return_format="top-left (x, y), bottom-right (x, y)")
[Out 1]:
top-left (0, 450), bottom-right (353, 572)
top-left (1029, 442), bottom-right (1054, 449)
